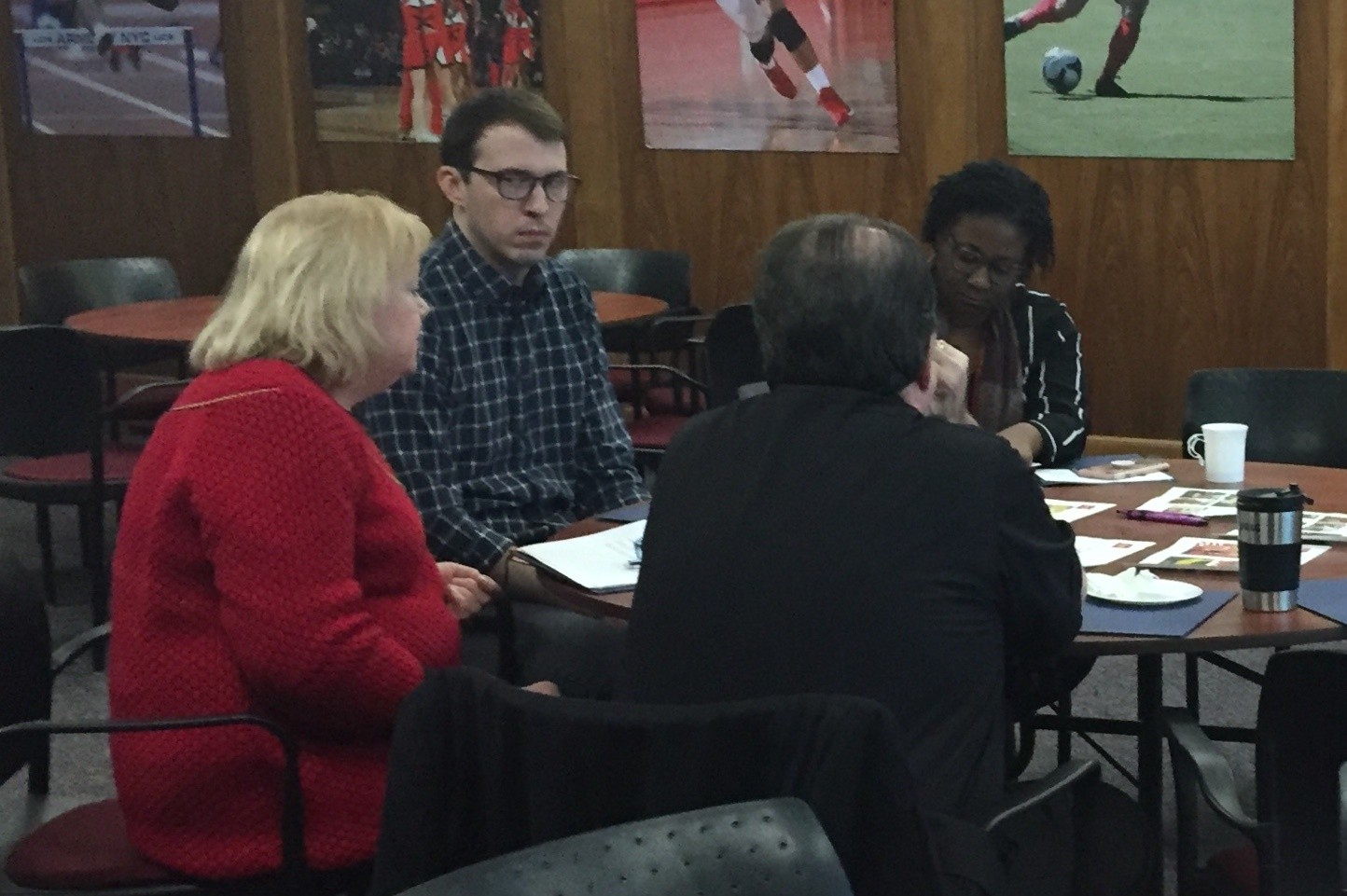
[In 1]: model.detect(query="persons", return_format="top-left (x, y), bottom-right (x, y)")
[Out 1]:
top-left (27, 0), bottom-right (115, 54)
top-left (1004, 0), bottom-right (1148, 97)
top-left (306, 0), bottom-right (538, 144)
top-left (621, 214), bottom-right (1163, 896)
top-left (109, 187), bottom-right (562, 896)
top-left (716, 0), bottom-right (855, 128)
top-left (919, 161), bottom-right (1089, 468)
top-left (350, 89), bottom-right (649, 700)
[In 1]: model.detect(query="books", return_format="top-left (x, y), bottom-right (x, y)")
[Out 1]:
top-left (513, 518), bottom-right (647, 593)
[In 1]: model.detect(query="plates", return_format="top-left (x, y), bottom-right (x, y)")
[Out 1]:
top-left (1086, 579), bottom-right (1203, 605)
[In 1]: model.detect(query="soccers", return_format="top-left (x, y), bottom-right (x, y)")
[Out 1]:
top-left (1042, 46), bottom-right (1083, 93)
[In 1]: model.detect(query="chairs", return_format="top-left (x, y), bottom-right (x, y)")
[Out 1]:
top-left (0, 253), bottom-right (370, 896)
top-left (1158, 643), bottom-right (1347, 896)
top-left (556, 246), bottom-right (762, 488)
top-left (1180, 363), bottom-right (1347, 464)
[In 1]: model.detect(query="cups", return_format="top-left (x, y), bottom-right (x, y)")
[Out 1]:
top-left (1188, 423), bottom-right (1249, 484)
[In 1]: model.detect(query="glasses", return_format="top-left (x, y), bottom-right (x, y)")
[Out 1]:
top-left (474, 167), bottom-right (581, 203)
top-left (944, 228), bottom-right (1020, 287)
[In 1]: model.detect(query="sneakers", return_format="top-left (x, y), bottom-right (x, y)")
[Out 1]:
top-left (759, 56), bottom-right (797, 99)
top-left (1095, 79), bottom-right (1129, 97)
top-left (817, 86), bottom-right (854, 127)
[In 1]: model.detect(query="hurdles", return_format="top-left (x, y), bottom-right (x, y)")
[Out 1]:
top-left (12, 24), bottom-right (204, 138)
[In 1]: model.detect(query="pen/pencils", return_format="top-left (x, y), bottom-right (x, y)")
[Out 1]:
top-left (1117, 510), bottom-right (1209, 526)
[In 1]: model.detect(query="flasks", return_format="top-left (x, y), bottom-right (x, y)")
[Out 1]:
top-left (1236, 482), bottom-right (1313, 612)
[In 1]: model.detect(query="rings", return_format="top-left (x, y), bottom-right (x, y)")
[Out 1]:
top-left (936, 340), bottom-right (948, 353)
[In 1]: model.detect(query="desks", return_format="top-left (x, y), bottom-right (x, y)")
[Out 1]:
top-left (538, 456), bottom-right (1347, 896)
top-left (61, 295), bottom-right (233, 523)
top-left (580, 288), bottom-right (666, 329)
